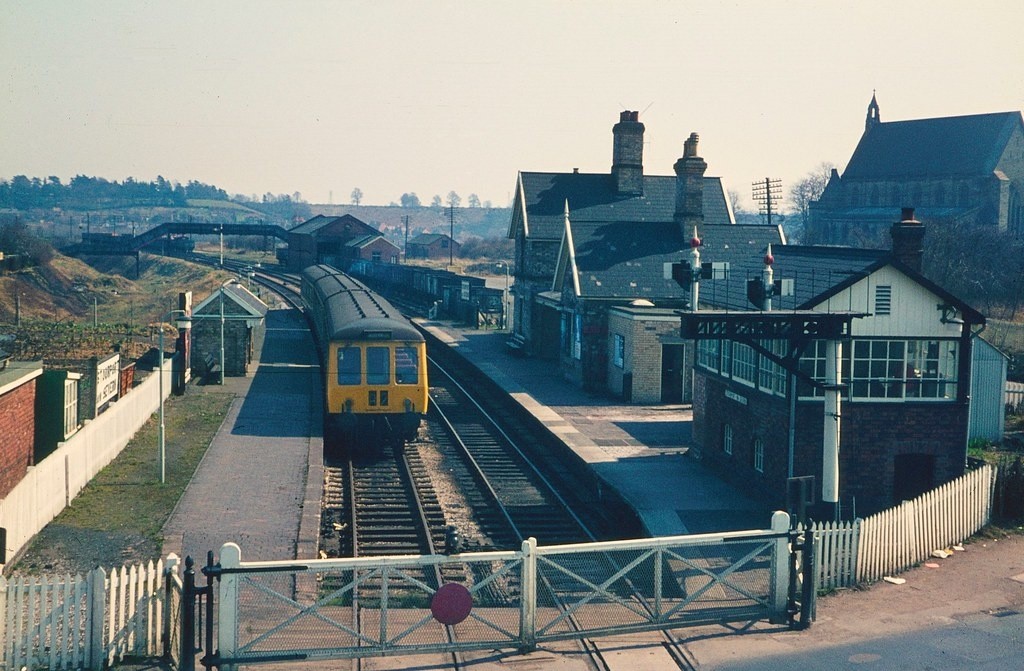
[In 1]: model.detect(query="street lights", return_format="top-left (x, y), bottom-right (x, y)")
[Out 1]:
top-left (219, 278), bottom-right (241, 385)
top-left (159, 308), bottom-right (191, 483)
top-left (248, 270), bottom-right (255, 291)
top-left (496, 259), bottom-right (509, 330)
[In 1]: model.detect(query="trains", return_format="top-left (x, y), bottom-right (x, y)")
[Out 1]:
top-left (303, 262), bottom-right (428, 451)
